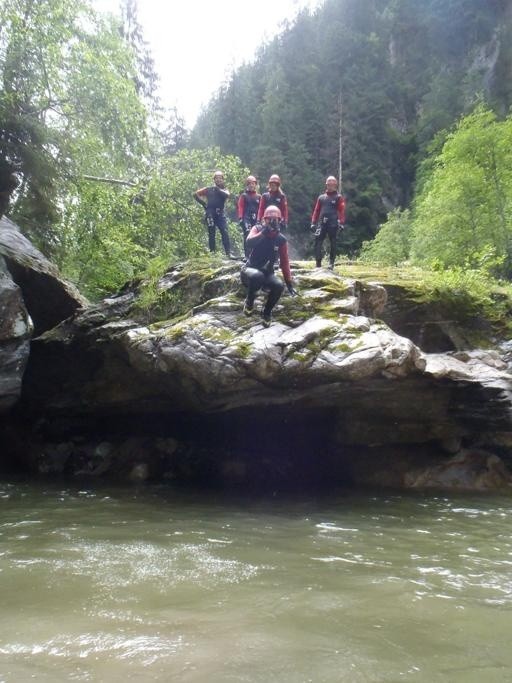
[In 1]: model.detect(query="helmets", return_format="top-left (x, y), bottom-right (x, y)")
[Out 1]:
top-left (264, 205), bottom-right (282, 218)
top-left (214, 172), bottom-right (223, 179)
top-left (246, 176), bottom-right (258, 185)
top-left (268, 173), bottom-right (281, 184)
top-left (326, 176), bottom-right (337, 186)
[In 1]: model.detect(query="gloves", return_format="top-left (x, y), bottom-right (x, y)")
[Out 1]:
top-left (286, 282), bottom-right (299, 296)
top-left (338, 224), bottom-right (344, 231)
top-left (310, 225), bottom-right (316, 233)
top-left (266, 220), bottom-right (276, 231)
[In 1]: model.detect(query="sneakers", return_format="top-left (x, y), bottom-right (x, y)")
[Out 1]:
top-left (243, 297), bottom-right (254, 316)
top-left (228, 253), bottom-right (238, 259)
top-left (261, 309), bottom-right (273, 328)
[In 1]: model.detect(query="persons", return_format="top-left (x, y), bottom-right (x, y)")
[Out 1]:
top-left (191, 169), bottom-right (234, 259)
top-left (239, 205), bottom-right (301, 329)
top-left (309, 175), bottom-right (347, 270)
top-left (238, 174), bottom-right (261, 262)
top-left (257, 173), bottom-right (289, 270)
